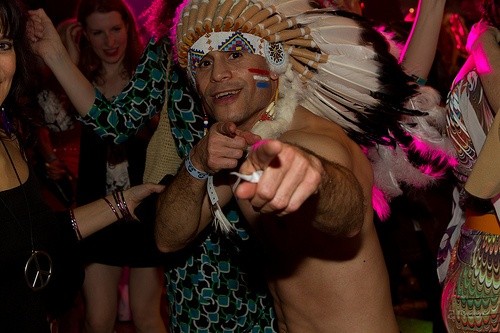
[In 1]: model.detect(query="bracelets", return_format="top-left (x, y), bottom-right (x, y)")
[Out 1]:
top-left (101, 188), bottom-right (134, 224)
top-left (69, 209), bottom-right (82, 241)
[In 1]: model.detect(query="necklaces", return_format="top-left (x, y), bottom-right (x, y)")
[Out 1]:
top-left (0, 135), bottom-right (52, 288)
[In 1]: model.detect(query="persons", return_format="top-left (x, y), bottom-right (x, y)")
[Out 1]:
top-left (1, 0), bottom-right (500, 332)
top-left (154, 1), bottom-right (399, 333)
top-left (0, 2), bottom-right (165, 331)
